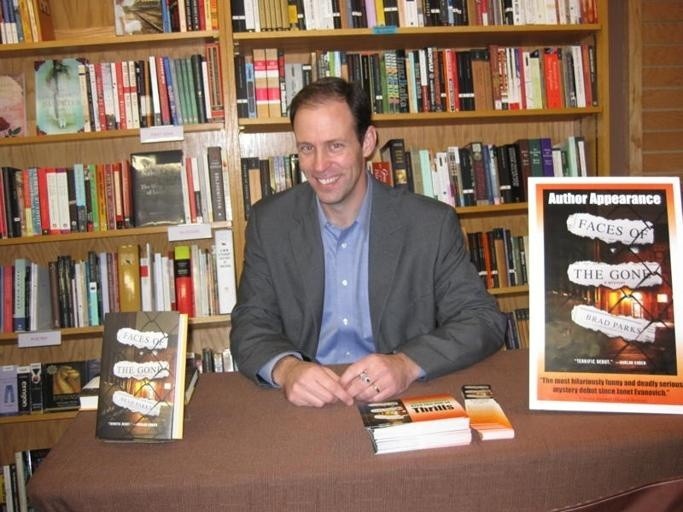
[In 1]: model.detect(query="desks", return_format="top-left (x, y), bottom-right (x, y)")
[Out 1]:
top-left (18, 341), bottom-right (682, 512)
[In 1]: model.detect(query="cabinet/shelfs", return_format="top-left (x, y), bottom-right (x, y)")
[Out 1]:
top-left (0, 1), bottom-right (238, 511)
top-left (223, 0), bottom-right (613, 373)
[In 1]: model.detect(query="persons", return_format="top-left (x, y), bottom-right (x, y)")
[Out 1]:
top-left (231, 76), bottom-right (507, 406)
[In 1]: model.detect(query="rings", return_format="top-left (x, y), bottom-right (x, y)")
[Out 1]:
top-left (372, 384), bottom-right (379, 392)
top-left (360, 372), bottom-right (371, 383)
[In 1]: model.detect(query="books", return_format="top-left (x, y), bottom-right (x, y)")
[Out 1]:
top-left (2, 360), bottom-right (103, 415)
top-left (114, 1), bottom-right (219, 36)
top-left (96, 312), bottom-right (199, 442)
top-left (460, 226), bottom-right (528, 290)
top-left (500, 308), bottom-right (529, 352)
top-left (0, 146), bottom-right (238, 334)
top-left (228, 1), bottom-right (599, 120)
top-left (240, 153), bottom-right (309, 222)
top-left (371, 136), bottom-right (588, 208)
top-left (193, 348), bottom-right (240, 372)
top-left (1, 449), bottom-right (52, 512)
top-left (1, 42), bottom-right (225, 137)
top-left (1, 1), bottom-right (55, 43)
